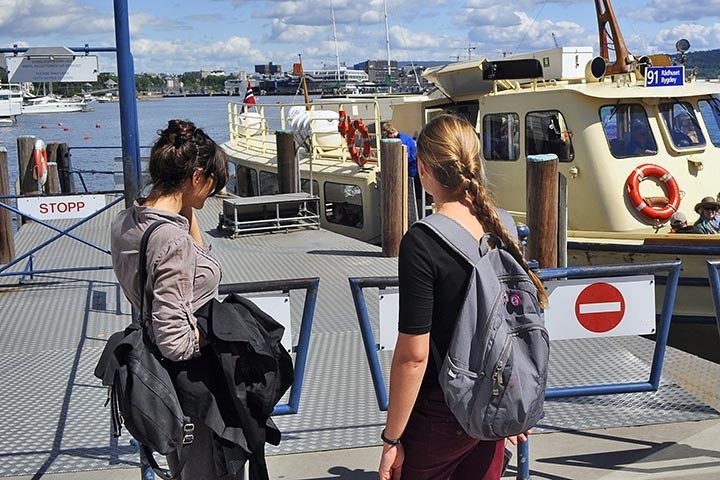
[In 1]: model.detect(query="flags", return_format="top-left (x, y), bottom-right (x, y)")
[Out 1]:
top-left (240, 81), bottom-right (256, 114)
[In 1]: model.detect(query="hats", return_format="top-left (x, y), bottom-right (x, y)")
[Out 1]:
top-left (670, 212), bottom-right (687, 225)
top-left (694, 197), bottom-right (720, 214)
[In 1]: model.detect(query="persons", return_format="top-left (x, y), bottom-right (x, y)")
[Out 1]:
top-left (627, 119), bottom-right (657, 154)
top-left (379, 115), bottom-right (549, 480)
top-left (382, 121), bottom-right (417, 195)
top-left (694, 197), bottom-right (720, 234)
top-left (112, 120), bottom-right (230, 480)
top-left (671, 112), bottom-right (700, 145)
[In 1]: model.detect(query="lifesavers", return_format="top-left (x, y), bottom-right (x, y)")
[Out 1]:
top-left (347, 119), bottom-right (371, 165)
top-left (628, 163), bottom-right (681, 221)
top-left (34, 139), bottom-right (47, 184)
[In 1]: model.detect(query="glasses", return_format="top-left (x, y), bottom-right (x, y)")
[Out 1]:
top-left (703, 206), bottom-right (718, 212)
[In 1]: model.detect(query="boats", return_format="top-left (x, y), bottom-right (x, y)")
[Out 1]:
top-left (221, 0), bottom-right (720, 364)
top-left (0, 83), bottom-right (24, 126)
top-left (22, 96), bottom-right (88, 114)
top-left (321, 0), bottom-right (443, 99)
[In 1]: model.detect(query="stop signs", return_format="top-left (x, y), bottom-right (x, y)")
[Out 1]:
top-left (574, 282), bottom-right (626, 334)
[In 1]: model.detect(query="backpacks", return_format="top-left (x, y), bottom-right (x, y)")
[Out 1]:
top-left (408, 207), bottom-right (549, 441)
top-left (94, 221), bottom-right (195, 480)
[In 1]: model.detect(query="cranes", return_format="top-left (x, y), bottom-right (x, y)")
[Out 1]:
top-left (444, 44), bottom-right (513, 63)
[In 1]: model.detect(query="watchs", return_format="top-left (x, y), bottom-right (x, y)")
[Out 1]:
top-left (381, 429), bottom-right (400, 444)
